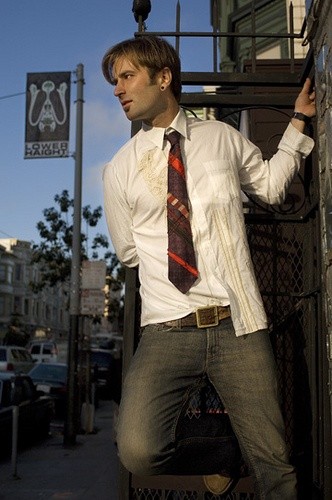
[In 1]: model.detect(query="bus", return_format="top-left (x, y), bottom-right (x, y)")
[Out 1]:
top-left (90, 333), bottom-right (125, 358)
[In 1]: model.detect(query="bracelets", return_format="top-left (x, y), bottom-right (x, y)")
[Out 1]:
top-left (293, 112), bottom-right (312, 125)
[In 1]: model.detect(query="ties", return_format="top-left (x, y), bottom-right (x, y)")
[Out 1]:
top-left (163, 131), bottom-right (199, 292)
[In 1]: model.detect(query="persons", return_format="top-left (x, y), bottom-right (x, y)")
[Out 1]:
top-left (101, 34), bottom-right (316, 500)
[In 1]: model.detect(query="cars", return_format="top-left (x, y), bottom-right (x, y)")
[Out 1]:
top-left (81, 348), bottom-right (119, 398)
top-left (0, 372), bottom-right (40, 450)
top-left (29, 363), bottom-right (97, 417)
top-left (29, 342), bottom-right (58, 364)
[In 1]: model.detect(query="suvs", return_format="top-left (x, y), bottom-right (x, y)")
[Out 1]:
top-left (0, 345), bottom-right (38, 374)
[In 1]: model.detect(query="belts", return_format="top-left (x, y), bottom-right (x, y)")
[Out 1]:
top-left (165, 305), bottom-right (230, 328)
